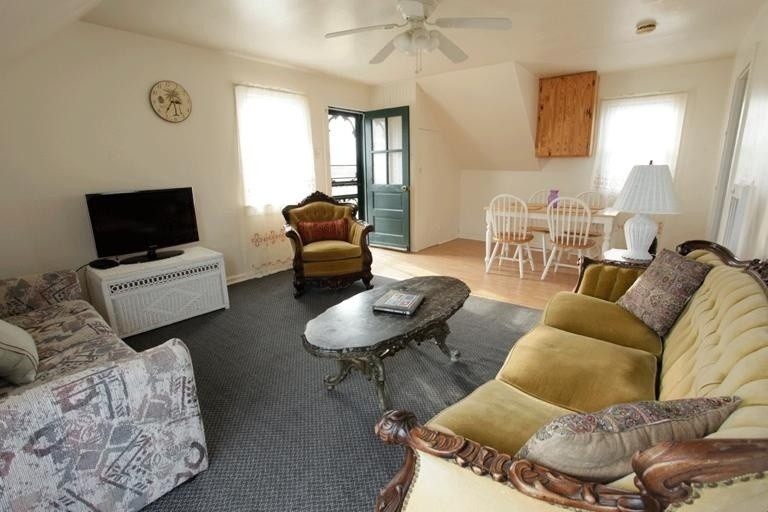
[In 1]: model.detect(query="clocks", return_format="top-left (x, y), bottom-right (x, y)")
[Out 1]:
top-left (150, 80), bottom-right (192, 122)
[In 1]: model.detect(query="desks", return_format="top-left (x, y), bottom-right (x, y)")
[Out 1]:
top-left (603, 247), bottom-right (654, 269)
top-left (483, 202), bottom-right (621, 273)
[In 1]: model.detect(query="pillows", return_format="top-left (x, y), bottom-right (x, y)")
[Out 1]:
top-left (615, 248), bottom-right (713, 337)
top-left (514, 396), bottom-right (741, 483)
top-left (0, 316), bottom-right (38, 384)
top-left (295, 220), bottom-right (350, 247)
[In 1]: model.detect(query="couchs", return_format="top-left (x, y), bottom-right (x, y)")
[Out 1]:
top-left (1, 268), bottom-right (209, 512)
top-left (373, 240), bottom-right (768, 512)
top-left (281, 191), bottom-right (373, 299)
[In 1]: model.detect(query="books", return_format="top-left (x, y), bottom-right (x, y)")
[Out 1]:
top-left (372, 289), bottom-right (426, 315)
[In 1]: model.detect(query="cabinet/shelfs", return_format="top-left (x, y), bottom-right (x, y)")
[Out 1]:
top-left (534, 70), bottom-right (599, 157)
top-left (86, 246), bottom-right (230, 338)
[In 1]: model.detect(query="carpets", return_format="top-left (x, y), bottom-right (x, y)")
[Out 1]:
top-left (0, 268), bottom-right (545, 512)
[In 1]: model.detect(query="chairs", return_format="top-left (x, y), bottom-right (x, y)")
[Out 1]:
top-left (486, 194), bottom-right (534, 279)
top-left (512, 189), bottom-right (551, 266)
top-left (564, 192), bottom-right (606, 260)
top-left (541, 197), bottom-right (596, 282)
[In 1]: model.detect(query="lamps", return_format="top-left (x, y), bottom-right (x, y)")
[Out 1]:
top-left (391, 28), bottom-right (439, 73)
top-left (609, 160), bottom-right (683, 261)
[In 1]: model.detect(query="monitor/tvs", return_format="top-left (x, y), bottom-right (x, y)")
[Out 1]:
top-left (85, 187), bottom-right (200, 265)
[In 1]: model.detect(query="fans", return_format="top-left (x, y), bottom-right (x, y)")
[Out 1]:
top-left (323, 1), bottom-right (510, 65)
